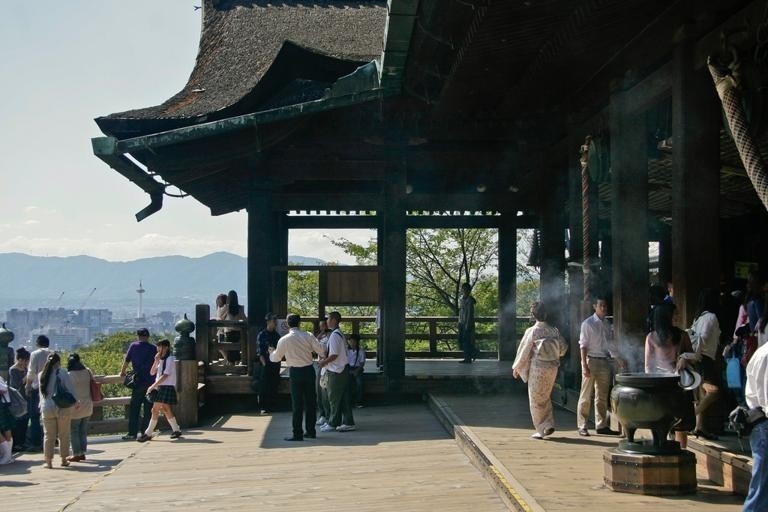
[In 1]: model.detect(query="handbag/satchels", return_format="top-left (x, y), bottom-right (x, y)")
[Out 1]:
top-left (724, 348), bottom-right (742, 389)
top-left (319, 366), bottom-right (329, 391)
top-left (52, 368), bottom-right (75, 409)
top-left (89, 381), bottom-right (106, 402)
top-left (123, 369), bottom-right (141, 390)
top-left (728, 405), bottom-right (764, 437)
top-left (740, 330), bottom-right (758, 366)
top-left (145, 389), bottom-right (159, 404)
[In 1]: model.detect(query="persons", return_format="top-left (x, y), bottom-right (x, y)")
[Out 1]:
top-left (512, 302), bottom-right (569, 439)
top-left (0, 334), bottom-right (94, 469)
top-left (121, 327), bottom-right (158, 440)
top-left (138, 339), bottom-right (182, 442)
top-left (576, 296), bottom-right (625, 436)
top-left (457, 283), bottom-right (481, 364)
top-left (215, 290), bottom-right (366, 441)
top-left (644, 281), bottom-right (768, 512)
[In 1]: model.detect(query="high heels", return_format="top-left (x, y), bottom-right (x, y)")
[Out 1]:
top-left (692, 425), bottom-right (719, 441)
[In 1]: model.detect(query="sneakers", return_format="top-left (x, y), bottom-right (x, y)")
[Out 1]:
top-left (170, 431), bottom-right (182, 439)
top-left (121, 433), bottom-right (137, 440)
top-left (543, 428), bottom-right (555, 436)
top-left (42, 454), bottom-right (86, 469)
top-left (137, 433), bottom-right (152, 442)
top-left (531, 432), bottom-right (543, 439)
top-left (335, 424), bottom-right (356, 432)
top-left (258, 408), bottom-right (271, 416)
top-left (316, 415), bottom-right (336, 432)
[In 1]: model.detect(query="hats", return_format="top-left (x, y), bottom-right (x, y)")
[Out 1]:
top-left (673, 368), bottom-right (702, 392)
top-left (264, 311), bottom-right (281, 321)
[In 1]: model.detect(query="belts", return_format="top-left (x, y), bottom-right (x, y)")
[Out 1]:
top-left (588, 355), bottom-right (607, 360)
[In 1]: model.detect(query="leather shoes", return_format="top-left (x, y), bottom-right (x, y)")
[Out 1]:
top-left (283, 435), bottom-right (303, 441)
top-left (303, 432), bottom-right (317, 438)
top-left (597, 427), bottom-right (621, 436)
top-left (578, 428), bottom-right (590, 437)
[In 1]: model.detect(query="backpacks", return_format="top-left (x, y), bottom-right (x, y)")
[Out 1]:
top-left (1, 385), bottom-right (30, 419)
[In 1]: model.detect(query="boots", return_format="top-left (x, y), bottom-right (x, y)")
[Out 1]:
top-left (0, 437), bottom-right (15, 466)
